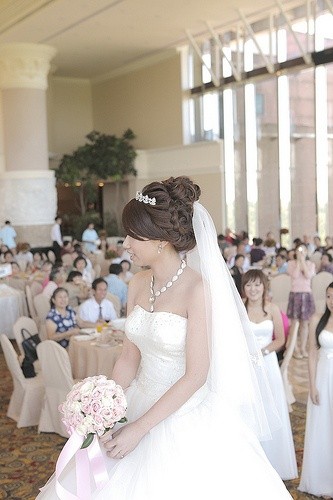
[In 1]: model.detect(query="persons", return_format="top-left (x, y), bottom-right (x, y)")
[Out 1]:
top-left (296, 281), bottom-right (333, 500)
top-left (78, 276), bottom-right (117, 323)
top-left (50, 215), bottom-right (64, 261)
top-left (82, 221), bottom-right (100, 254)
top-left (0, 220), bottom-right (17, 249)
top-left (287, 243), bottom-right (315, 359)
top-left (0, 230), bottom-right (134, 318)
top-left (242, 270), bottom-right (298, 481)
top-left (217, 228), bottom-right (333, 364)
top-left (35, 176), bottom-right (292, 500)
top-left (45, 287), bottom-right (104, 348)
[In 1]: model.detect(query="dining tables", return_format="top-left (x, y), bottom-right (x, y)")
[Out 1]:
top-left (67, 327), bottom-right (125, 380)
top-left (0, 284), bottom-right (26, 339)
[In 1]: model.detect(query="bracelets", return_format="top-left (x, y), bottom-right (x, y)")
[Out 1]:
top-left (264, 347), bottom-right (269, 355)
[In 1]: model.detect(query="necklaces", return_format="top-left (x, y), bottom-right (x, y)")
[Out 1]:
top-left (149, 259), bottom-right (186, 313)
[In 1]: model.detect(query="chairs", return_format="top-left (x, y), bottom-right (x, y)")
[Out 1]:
top-left (0, 245), bottom-right (333, 438)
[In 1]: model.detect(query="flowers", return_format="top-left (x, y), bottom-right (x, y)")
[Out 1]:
top-left (58, 375), bottom-right (129, 449)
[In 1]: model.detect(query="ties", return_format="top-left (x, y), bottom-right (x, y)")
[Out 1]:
top-left (100, 307), bottom-right (103, 320)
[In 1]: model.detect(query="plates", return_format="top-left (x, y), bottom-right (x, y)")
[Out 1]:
top-left (89, 340), bottom-right (118, 348)
top-left (75, 335), bottom-right (96, 342)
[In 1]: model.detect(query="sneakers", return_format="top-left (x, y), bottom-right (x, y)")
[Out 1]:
top-left (301, 351), bottom-right (309, 357)
top-left (292, 346), bottom-right (302, 359)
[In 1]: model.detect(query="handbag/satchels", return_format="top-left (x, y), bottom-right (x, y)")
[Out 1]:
top-left (21, 328), bottom-right (41, 362)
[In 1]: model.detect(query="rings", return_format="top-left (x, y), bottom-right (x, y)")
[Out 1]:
top-left (119, 451), bottom-right (124, 457)
